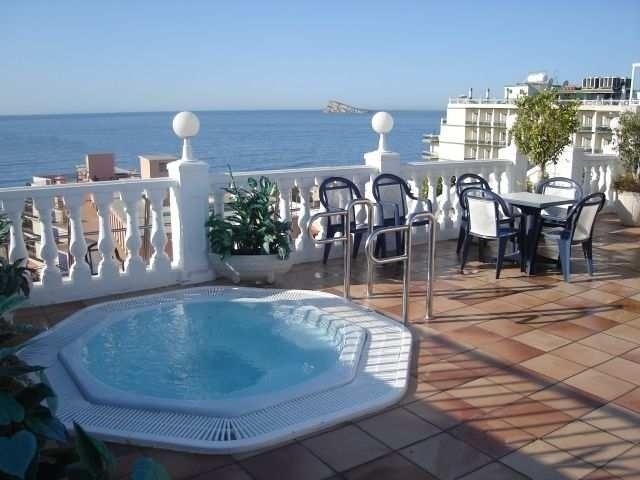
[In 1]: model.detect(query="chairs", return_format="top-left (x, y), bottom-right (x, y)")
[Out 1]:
top-left (318, 173), bottom-right (434, 265)
top-left (453, 172), bottom-right (608, 283)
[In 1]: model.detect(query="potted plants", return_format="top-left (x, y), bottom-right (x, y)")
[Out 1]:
top-left (203, 174), bottom-right (296, 286)
top-left (608, 108), bottom-right (640, 227)
